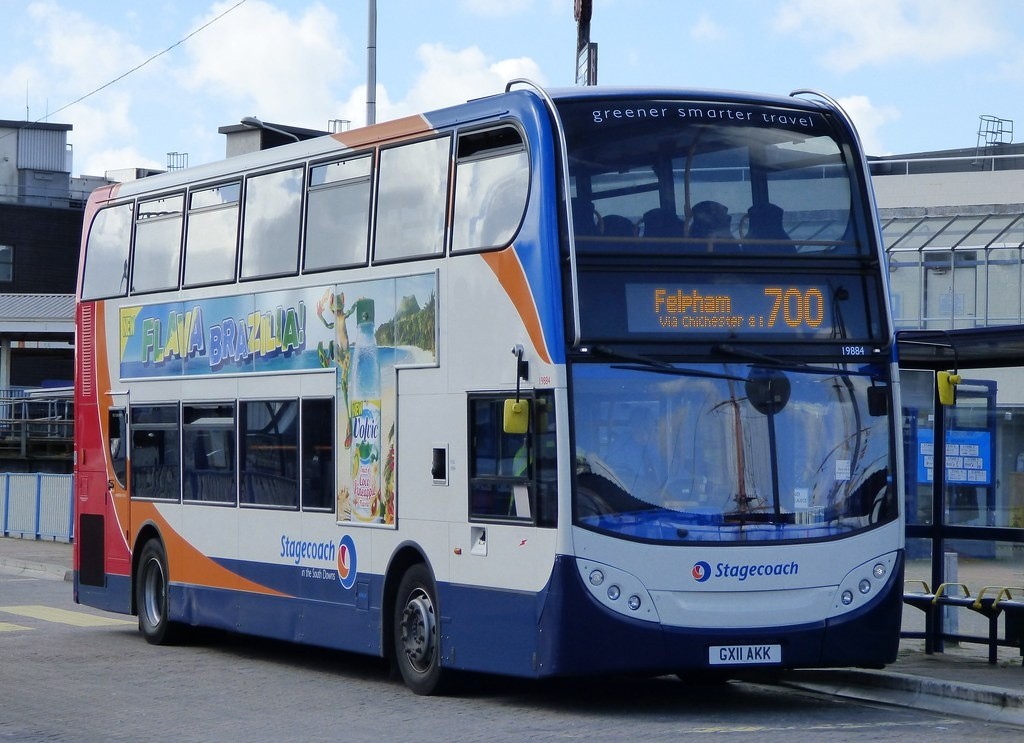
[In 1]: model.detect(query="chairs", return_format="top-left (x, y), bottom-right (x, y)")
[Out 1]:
top-left (565, 196), bottom-right (799, 254)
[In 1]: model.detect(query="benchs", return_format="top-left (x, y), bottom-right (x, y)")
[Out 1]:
top-left (903, 580), bottom-right (1024, 664)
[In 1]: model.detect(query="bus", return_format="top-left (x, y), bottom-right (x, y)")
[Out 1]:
top-left (68, 77), bottom-right (962, 697)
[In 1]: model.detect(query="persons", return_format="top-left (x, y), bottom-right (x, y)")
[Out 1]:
top-left (510, 406), bottom-right (666, 517)
